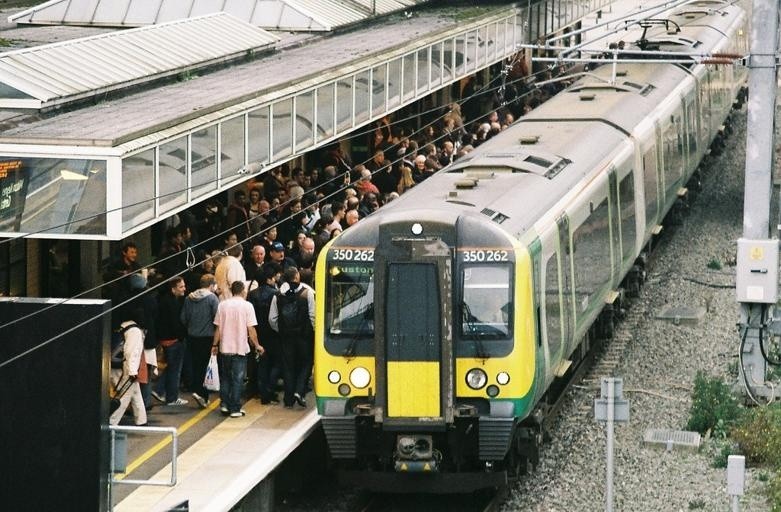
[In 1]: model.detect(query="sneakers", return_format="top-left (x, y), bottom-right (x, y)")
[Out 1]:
top-left (191, 391), bottom-right (208, 409)
top-left (294, 392), bottom-right (307, 409)
top-left (230, 411), bottom-right (245, 418)
top-left (167, 397), bottom-right (189, 407)
top-left (150, 390), bottom-right (167, 403)
top-left (221, 407), bottom-right (230, 416)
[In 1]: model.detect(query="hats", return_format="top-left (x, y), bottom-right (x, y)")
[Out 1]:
top-left (269, 241), bottom-right (285, 251)
top-left (130, 274), bottom-right (145, 289)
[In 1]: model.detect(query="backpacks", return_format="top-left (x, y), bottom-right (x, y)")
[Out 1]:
top-left (108, 324), bottom-right (140, 370)
top-left (275, 286), bottom-right (310, 334)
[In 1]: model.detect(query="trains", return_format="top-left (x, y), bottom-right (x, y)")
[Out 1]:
top-left (311, 0), bottom-right (754, 502)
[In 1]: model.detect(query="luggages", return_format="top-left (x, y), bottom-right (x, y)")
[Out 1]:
top-left (105, 376), bottom-right (137, 415)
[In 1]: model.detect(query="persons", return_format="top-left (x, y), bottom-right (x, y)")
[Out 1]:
top-left (371, 76), bottom-right (514, 198)
top-left (109, 165), bottom-right (382, 428)
top-left (583, 41), bottom-right (625, 73)
top-left (496, 63), bottom-right (571, 112)
top-left (48, 243), bottom-right (62, 295)
